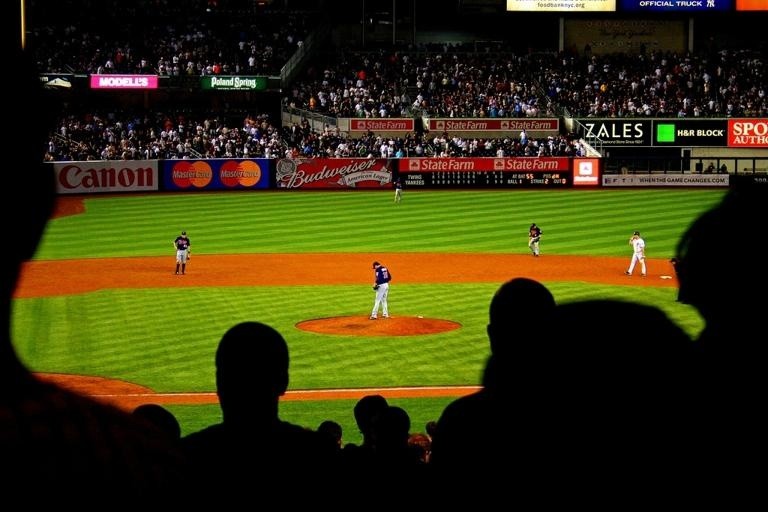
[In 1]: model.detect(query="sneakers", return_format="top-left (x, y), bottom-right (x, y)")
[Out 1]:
top-left (369, 316), bottom-right (389, 320)
top-left (641, 273), bottom-right (646, 276)
top-left (623, 271), bottom-right (632, 275)
top-left (175, 271), bottom-right (184, 275)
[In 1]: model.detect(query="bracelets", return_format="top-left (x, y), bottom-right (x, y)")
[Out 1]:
top-left (187, 251), bottom-right (190, 254)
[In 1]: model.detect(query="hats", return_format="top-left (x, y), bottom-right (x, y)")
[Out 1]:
top-left (182, 231), bottom-right (186, 235)
top-left (530, 223), bottom-right (535, 227)
top-left (372, 261), bottom-right (380, 269)
top-left (633, 231), bottom-right (639, 235)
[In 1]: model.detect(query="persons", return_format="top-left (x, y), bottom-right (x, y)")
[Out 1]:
top-left (0, 71), bottom-right (168, 510)
top-left (173, 231), bottom-right (191, 275)
top-left (129, 395), bottom-right (440, 512)
top-left (393, 177), bottom-right (402, 204)
top-left (28, 15), bottom-right (768, 158)
top-left (369, 262), bottom-right (392, 320)
top-left (437, 177), bottom-right (768, 512)
top-left (168, 318), bottom-right (347, 509)
